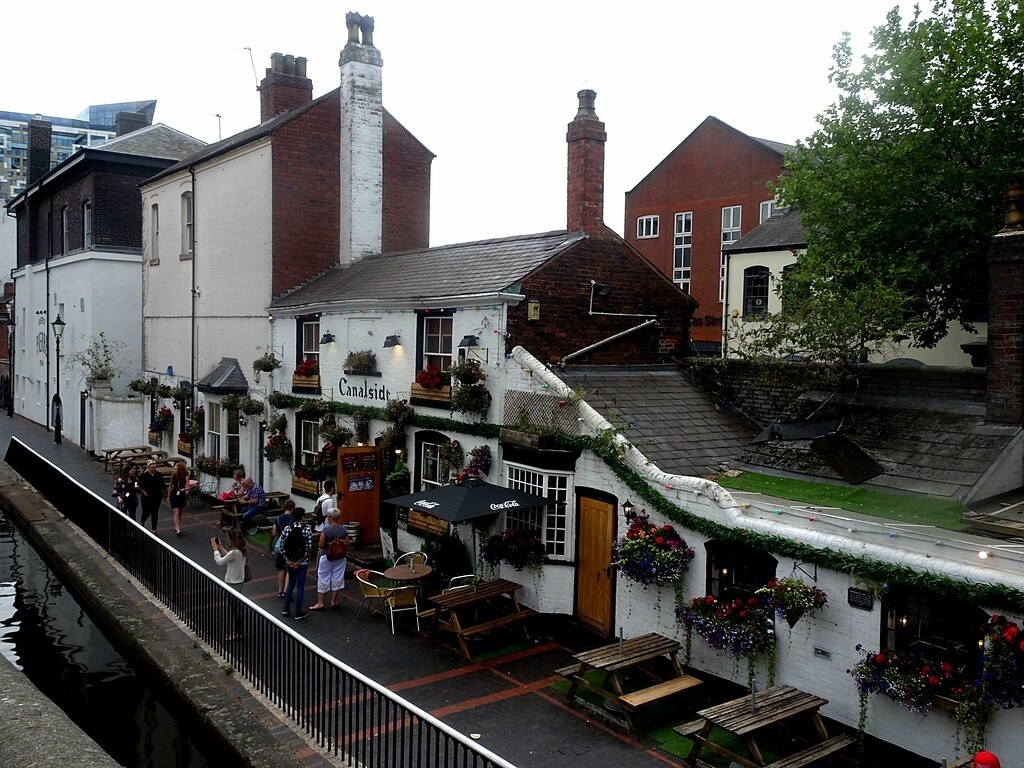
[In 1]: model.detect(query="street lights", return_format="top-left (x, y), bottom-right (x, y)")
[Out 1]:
top-left (4, 319), bottom-right (16, 417)
top-left (50, 313), bottom-right (67, 445)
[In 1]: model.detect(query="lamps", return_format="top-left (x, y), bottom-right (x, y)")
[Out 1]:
top-left (458, 335), bottom-right (479, 346)
top-left (319, 334), bottom-right (334, 343)
top-left (383, 335), bottom-right (401, 347)
top-left (622, 499), bottom-right (649, 525)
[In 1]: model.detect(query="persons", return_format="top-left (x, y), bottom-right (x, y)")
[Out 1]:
top-left (279, 507), bottom-right (312, 620)
top-left (273, 500), bottom-right (295, 599)
top-left (113, 466), bottom-right (146, 521)
top-left (314, 480), bottom-right (335, 532)
top-left (210, 528), bottom-right (246, 641)
top-left (309, 508), bottom-right (350, 611)
top-left (166, 462), bottom-right (189, 535)
top-left (139, 460), bottom-right (166, 535)
top-left (217, 470), bottom-right (265, 536)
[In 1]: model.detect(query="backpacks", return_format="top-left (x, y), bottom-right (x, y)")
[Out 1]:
top-left (326, 524), bottom-right (347, 561)
top-left (283, 524), bottom-right (306, 560)
top-left (270, 516), bottom-right (293, 555)
top-left (311, 498), bottom-right (329, 525)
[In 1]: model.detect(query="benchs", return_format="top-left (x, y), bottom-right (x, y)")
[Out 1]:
top-left (416, 607), bottom-right (448, 618)
top-left (762, 733), bottom-right (860, 768)
top-left (555, 663), bottom-right (592, 678)
top-left (457, 609), bottom-right (536, 637)
top-left (210, 504), bottom-right (286, 519)
top-left (673, 718), bottom-right (710, 737)
top-left (100, 457), bottom-right (165, 471)
top-left (618, 675), bottom-right (704, 712)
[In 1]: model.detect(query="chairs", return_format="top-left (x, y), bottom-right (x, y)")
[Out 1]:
top-left (355, 551), bottom-right (480, 634)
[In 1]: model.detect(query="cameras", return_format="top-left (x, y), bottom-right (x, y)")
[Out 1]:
top-left (209, 537), bottom-right (219, 544)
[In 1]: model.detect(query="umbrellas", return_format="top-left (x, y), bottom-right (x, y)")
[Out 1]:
top-left (381, 480), bottom-right (567, 590)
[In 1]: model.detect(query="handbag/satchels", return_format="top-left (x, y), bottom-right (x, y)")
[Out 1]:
top-left (243, 556), bottom-right (252, 582)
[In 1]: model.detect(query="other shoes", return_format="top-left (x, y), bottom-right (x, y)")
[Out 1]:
top-left (282, 608), bottom-right (292, 614)
top-left (249, 526), bottom-right (258, 535)
top-left (330, 602), bottom-right (338, 608)
top-left (294, 611), bottom-right (309, 619)
top-left (309, 603), bottom-right (324, 609)
top-left (278, 591), bottom-right (285, 597)
top-left (152, 529), bottom-right (158, 536)
top-left (174, 528), bottom-right (181, 535)
top-left (225, 632), bottom-right (242, 641)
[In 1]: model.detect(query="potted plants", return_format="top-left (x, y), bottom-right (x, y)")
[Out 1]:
top-left (220, 393), bottom-right (264, 416)
top-left (253, 355), bottom-right (282, 374)
top-left (451, 355), bottom-right (486, 387)
top-left (128, 380), bottom-right (188, 400)
top-left (500, 425), bottom-right (555, 449)
top-left (343, 351), bottom-right (376, 377)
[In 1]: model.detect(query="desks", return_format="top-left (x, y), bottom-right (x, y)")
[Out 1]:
top-left (220, 492), bottom-right (290, 532)
top-left (566, 634), bottom-right (684, 732)
top-left (688, 686), bottom-right (828, 768)
top-left (384, 564), bottom-right (432, 622)
top-left (428, 578), bottom-right (529, 660)
top-left (102, 447), bottom-right (196, 475)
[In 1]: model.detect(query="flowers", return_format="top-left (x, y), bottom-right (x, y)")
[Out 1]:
top-left (417, 365), bottom-right (444, 388)
top-left (481, 529), bottom-right (548, 570)
top-left (196, 454), bottom-right (238, 477)
top-left (440, 440), bottom-right (463, 477)
top-left (159, 405), bottom-right (171, 416)
top-left (847, 614), bottom-right (1024, 758)
top-left (149, 421), bottom-right (162, 432)
top-left (608, 513), bottom-right (694, 607)
top-left (468, 446), bottom-right (492, 476)
top-left (676, 594), bottom-right (776, 693)
top-left (759, 578), bottom-right (826, 618)
top-left (179, 433), bottom-right (190, 444)
top-left (293, 360), bottom-right (318, 377)
top-left (456, 469), bottom-right (480, 485)
top-left (263, 434), bottom-right (334, 482)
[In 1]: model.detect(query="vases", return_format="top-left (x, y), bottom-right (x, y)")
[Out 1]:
top-left (785, 608), bottom-right (804, 629)
top-left (479, 461), bottom-right (491, 476)
top-left (445, 456), bottom-right (459, 470)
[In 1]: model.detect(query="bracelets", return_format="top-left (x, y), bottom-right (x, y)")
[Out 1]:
top-left (167, 496), bottom-right (170, 497)
top-left (213, 548), bottom-right (218, 551)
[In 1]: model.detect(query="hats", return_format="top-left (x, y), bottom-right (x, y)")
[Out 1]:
top-left (147, 459), bottom-right (156, 468)
